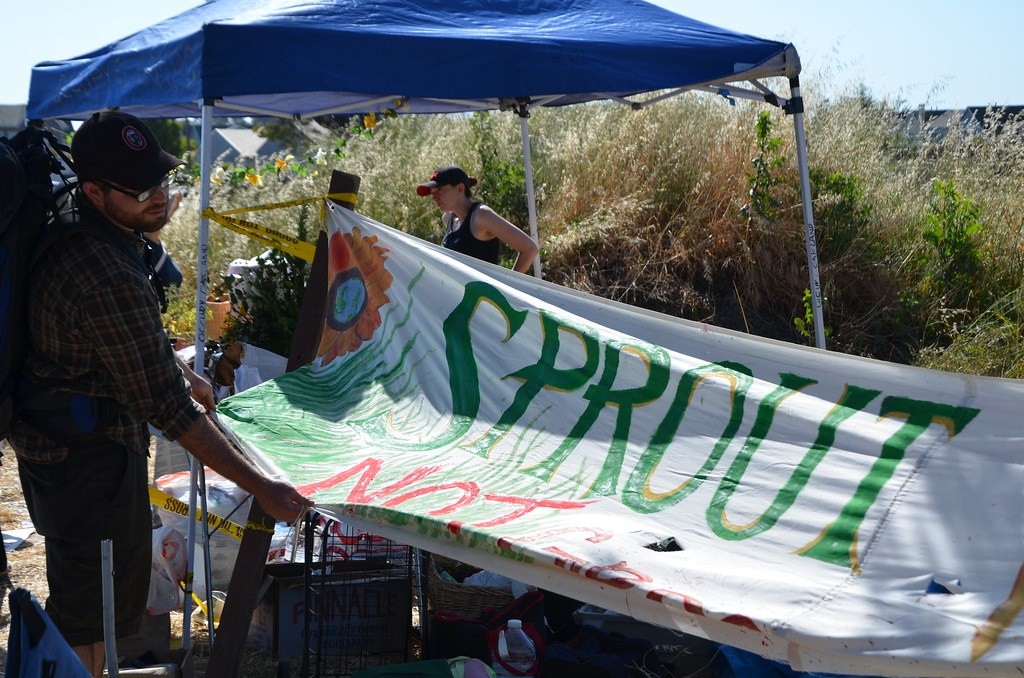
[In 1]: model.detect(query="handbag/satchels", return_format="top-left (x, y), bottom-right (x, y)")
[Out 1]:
top-left (5, 589), bottom-right (93, 678)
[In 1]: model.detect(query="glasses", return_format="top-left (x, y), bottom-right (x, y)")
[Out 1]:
top-left (86, 168), bottom-right (178, 204)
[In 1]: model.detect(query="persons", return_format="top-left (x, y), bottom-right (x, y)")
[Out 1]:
top-left (10, 107), bottom-right (311, 678)
top-left (417, 165), bottom-right (538, 274)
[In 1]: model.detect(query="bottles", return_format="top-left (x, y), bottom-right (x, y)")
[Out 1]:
top-left (438, 567), bottom-right (460, 583)
top-left (276, 661), bottom-right (290, 678)
top-left (492, 619), bottom-right (537, 678)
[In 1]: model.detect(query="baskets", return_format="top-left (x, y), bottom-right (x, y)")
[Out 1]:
top-left (413, 547), bottom-right (517, 620)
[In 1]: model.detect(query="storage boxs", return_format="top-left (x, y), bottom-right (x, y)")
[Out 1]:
top-left (255, 560), bottom-right (410, 658)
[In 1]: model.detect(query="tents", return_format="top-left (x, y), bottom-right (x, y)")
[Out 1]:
top-left (32, 0), bottom-right (827, 670)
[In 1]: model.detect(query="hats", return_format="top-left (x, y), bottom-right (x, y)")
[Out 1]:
top-left (70, 111), bottom-right (189, 191)
top-left (416, 166), bottom-right (472, 197)
